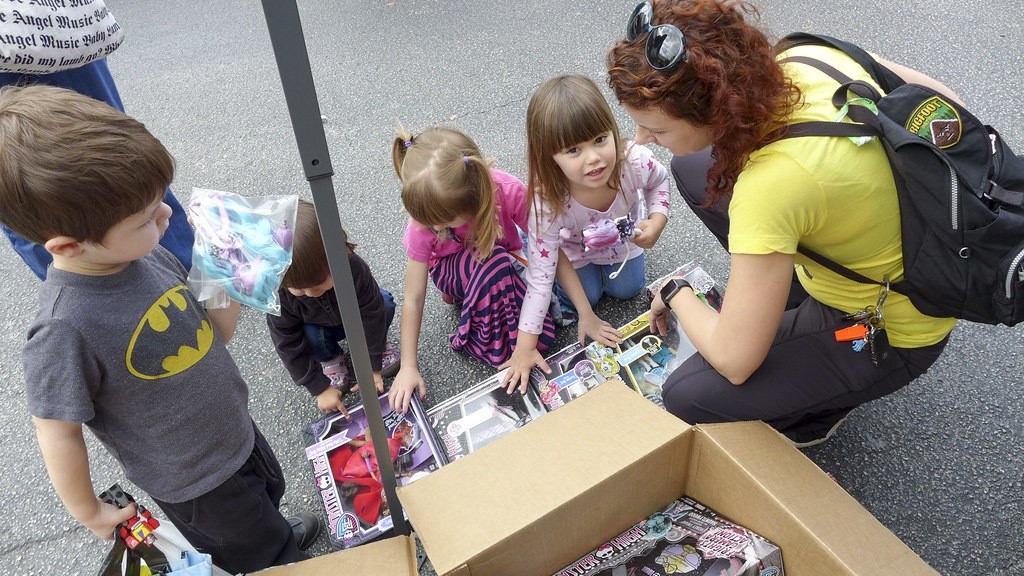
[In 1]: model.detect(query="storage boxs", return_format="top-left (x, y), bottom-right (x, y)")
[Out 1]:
top-left (300, 389), bottom-right (448, 551)
top-left (528, 335), bottom-right (636, 412)
top-left (245, 378), bottom-right (942, 576)
top-left (614, 307), bottom-right (698, 399)
top-left (550, 494), bottom-right (785, 576)
top-left (425, 366), bottom-right (549, 462)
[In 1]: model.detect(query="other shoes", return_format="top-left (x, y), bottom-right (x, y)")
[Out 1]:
top-left (286, 511), bottom-right (320, 551)
top-left (780, 404), bottom-right (852, 448)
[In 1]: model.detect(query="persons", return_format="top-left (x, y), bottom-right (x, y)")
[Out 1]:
top-left (3, 82), bottom-right (327, 571)
top-left (385, 121), bottom-right (624, 415)
top-left (497, 72), bottom-right (671, 392)
top-left (0, 0), bottom-right (210, 295)
top-left (607, 0), bottom-right (970, 447)
top-left (265, 196), bottom-right (398, 419)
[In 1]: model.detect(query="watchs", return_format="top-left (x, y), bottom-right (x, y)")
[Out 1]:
top-left (658, 277), bottom-right (696, 311)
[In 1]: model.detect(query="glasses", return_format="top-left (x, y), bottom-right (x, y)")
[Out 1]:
top-left (626, 1), bottom-right (686, 71)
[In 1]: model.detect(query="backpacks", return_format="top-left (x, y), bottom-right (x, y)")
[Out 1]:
top-left (753, 30), bottom-right (1024, 327)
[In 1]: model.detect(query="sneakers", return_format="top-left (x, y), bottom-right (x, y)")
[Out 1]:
top-left (380, 340), bottom-right (399, 377)
top-left (319, 349), bottom-right (350, 394)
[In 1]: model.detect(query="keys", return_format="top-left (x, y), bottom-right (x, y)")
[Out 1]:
top-left (834, 304), bottom-right (894, 367)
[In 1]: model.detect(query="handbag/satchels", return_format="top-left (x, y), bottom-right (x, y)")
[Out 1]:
top-left (0, 0), bottom-right (125, 75)
top-left (96, 483), bottom-right (238, 576)
